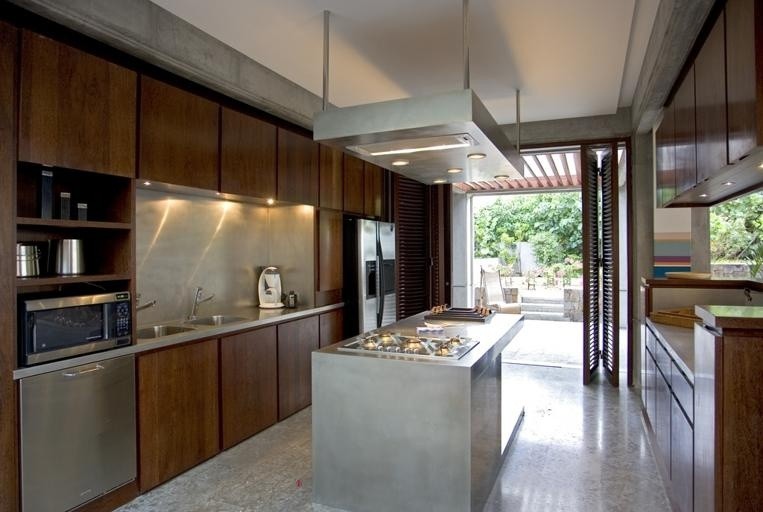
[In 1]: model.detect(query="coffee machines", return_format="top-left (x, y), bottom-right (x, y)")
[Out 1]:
top-left (257, 264), bottom-right (287, 310)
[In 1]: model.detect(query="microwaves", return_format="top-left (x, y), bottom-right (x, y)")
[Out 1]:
top-left (18, 290), bottom-right (132, 367)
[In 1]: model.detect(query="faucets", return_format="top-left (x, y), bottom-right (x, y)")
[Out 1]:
top-left (136, 293), bottom-right (157, 313)
top-left (191, 288), bottom-right (215, 318)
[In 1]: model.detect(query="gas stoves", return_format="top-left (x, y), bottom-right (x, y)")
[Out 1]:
top-left (338, 332), bottom-right (480, 361)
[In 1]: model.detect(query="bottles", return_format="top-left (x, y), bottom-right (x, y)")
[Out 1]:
top-left (288, 291), bottom-right (297, 309)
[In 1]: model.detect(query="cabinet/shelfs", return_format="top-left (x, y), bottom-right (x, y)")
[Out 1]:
top-left (655, 101), bottom-right (675, 208)
top-left (644, 327), bottom-right (657, 433)
top-left (15, 161), bottom-right (133, 369)
top-left (138, 339), bottom-right (220, 493)
top-left (15, 30), bottom-right (137, 177)
top-left (137, 75), bottom-right (220, 192)
top-left (671, 361), bottom-right (694, 512)
top-left (673, 64), bottom-right (694, 198)
top-left (655, 340), bottom-right (671, 479)
top-left (694, 11), bottom-right (727, 184)
top-left (692, 304), bottom-right (723, 512)
top-left (725, 1), bottom-right (760, 163)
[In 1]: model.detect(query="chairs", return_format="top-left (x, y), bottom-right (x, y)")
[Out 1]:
top-left (479, 266), bottom-right (521, 314)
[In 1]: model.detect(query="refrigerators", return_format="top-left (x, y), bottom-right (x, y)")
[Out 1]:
top-left (342, 214), bottom-right (399, 340)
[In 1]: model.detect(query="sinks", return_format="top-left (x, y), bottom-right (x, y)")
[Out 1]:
top-left (136, 326), bottom-right (196, 338)
top-left (185, 316), bottom-right (248, 328)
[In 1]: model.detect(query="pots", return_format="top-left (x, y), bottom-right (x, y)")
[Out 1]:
top-left (16, 242), bottom-right (42, 277)
top-left (44, 239), bottom-right (87, 275)
